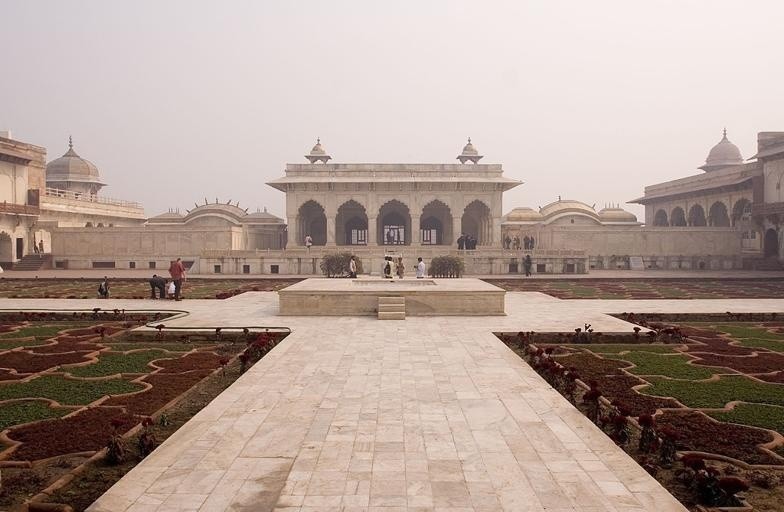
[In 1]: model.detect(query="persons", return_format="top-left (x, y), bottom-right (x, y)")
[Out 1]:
top-left (37, 240), bottom-right (45, 259)
top-left (149, 274), bottom-right (157, 299)
top-left (456, 235), bottom-right (477, 251)
top-left (413, 257), bottom-right (425, 279)
top-left (523, 254), bottom-right (532, 277)
top-left (349, 256), bottom-right (356, 277)
top-left (168, 257), bottom-right (185, 302)
top-left (382, 256), bottom-right (405, 280)
top-left (305, 234), bottom-right (312, 254)
top-left (33, 241), bottom-right (39, 257)
top-left (504, 234), bottom-right (535, 251)
top-left (155, 278), bottom-right (168, 298)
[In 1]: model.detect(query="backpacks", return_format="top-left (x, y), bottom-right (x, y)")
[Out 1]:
top-left (384, 262), bottom-right (392, 275)
